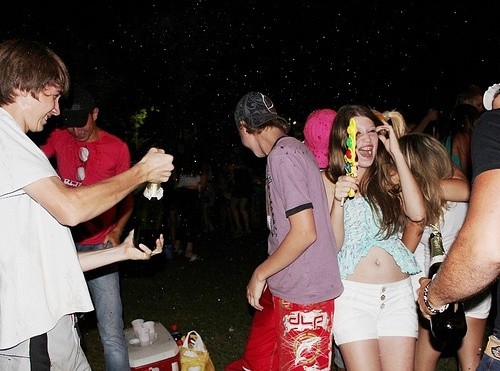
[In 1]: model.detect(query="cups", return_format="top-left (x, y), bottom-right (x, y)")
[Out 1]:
top-left (132, 319), bottom-right (155, 347)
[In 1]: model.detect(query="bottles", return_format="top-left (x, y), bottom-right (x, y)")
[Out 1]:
top-left (427, 231), bottom-right (467, 340)
top-left (133, 149), bottom-right (164, 252)
top-left (170, 325), bottom-right (182, 341)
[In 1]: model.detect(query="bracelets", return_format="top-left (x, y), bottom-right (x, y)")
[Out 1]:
top-left (424, 280), bottom-right (449, 314)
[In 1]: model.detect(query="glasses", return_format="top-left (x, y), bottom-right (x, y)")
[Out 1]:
top-left (75, 146), bottom-right (90, 182)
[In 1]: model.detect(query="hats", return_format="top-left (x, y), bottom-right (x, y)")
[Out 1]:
top-left (234, 92), bottom-right (290, 128)
top-left (58, 89), bottom-right (95, 131)
top-left (304, 109), bottom-right (338, 168)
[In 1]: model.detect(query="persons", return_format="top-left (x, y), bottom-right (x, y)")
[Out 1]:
top-left (0, 41), bottom-right (500, 371)
